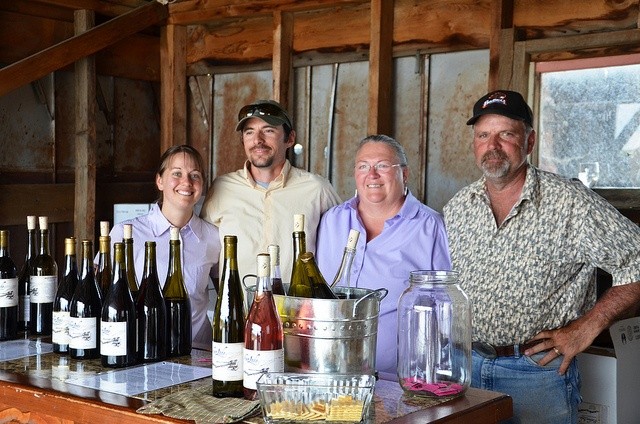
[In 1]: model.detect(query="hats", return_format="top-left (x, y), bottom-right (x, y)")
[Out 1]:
top-left (235, 100), bottom-right (293, 132)
top-left (466, 90), bottom-right (534, 129)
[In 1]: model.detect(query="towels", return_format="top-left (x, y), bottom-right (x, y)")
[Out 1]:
top-left (135, 386), bottom-right (261, 423)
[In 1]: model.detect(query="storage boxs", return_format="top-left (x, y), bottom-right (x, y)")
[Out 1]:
top-left (575, 315), bottom-right (640, 424)
top-left (256, 371), bottom-right (378, 424)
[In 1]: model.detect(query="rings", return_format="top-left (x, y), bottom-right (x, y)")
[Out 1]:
top-left (553, 347), bottom-right (561, 356)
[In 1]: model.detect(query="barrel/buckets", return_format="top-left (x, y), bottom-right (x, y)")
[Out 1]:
top-left (242, 273), bottom-right (388, 374)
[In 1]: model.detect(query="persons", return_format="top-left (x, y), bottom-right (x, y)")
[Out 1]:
top-left (199, 100), bottom-right (343, 311)
top-left (92, 145), bottom-right (220, 347)
top-left (315, 134), bottom-right (453, 375)
top-left (441, 89), bottom-right (640, 424)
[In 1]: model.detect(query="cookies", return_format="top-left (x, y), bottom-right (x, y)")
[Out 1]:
top-left (261, 394), bottom-right (365, 423)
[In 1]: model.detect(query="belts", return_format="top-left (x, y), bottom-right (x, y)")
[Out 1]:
top-left (454, 338), bottom-right (553, 359)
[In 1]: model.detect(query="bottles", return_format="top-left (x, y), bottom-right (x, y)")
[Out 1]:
top-left (51, 237), bottom-right (80, 354)
top-left (50, 355), bottom-right (66, 393)
top-left (0, 231), bottom-right (19, 341)
top-left (243, 253), bottom-right (285, 400)
top-left (329, 229), bottom-right (360, 299)
top-left (300, 252), bottom-right (339, 299)
top-left (287, 214), bottom-right (315, 298)
top-left (98, 369), bottom-right (135, 411)
top-left (96, 222), bottom-right (114, 298)
top-left (27, 338), bottom-right (50, 390)
top-left (135, 241), bottom-right (167, 361)
top-left (100, 242), bottom-right (138, 367)
top-left (69, 240), bottom-right (104, 360)
top-left (211, 235), bottom-right (246, 398)
top-left (66, 360), bottom-right (96, 398)
top-left (30, 216), bottom-right (59, 335)
top-left (396, 270), bottom-right (472, 398)
top-left (19, 215), bottom-right (38, 331)
top-left (163, 227), bottom-right (192, 359)
top-left (267, 244), bottom-right (285, 296)
top-left (122, 224), bottom-right (140, 304)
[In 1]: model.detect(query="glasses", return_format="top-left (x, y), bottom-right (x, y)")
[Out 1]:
top-left (355, 160), bottom-right (404, 173)
top-left (238, 103), bottom-right (291, 128)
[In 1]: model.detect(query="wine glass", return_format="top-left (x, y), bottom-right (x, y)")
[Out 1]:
top-left (578, 162), bottom-right (600, 189)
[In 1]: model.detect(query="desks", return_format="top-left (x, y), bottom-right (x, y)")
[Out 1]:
top-left (0, 333), bottom-right (512, 423)
